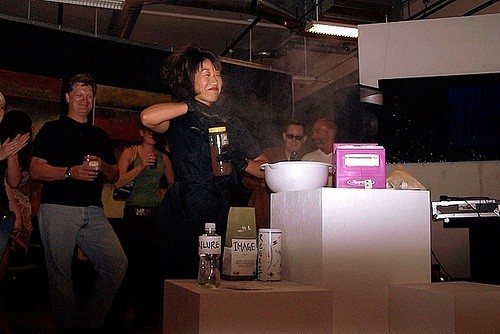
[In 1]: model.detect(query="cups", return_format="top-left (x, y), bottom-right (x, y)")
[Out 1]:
top-left (257, 228), bottom-right (283, 282)
top-left (82, 155), bottom-right (101, 178)
top-left (148, 152), bottom-right (158, 170)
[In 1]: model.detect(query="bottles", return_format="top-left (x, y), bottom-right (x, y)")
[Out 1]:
top-left (198, 222), bottom-right (222, 289)
top-left (208, 126), bottom-right (233, 177)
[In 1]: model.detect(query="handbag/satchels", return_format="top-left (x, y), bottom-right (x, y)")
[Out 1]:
top-left (113, 146), bottom-right (135, 201)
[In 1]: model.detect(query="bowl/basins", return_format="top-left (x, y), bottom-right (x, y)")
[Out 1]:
top-left (259, 161), bottom-right (334, 193)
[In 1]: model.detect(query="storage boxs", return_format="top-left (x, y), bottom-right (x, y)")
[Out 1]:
top-left (431, 199), bottom-right (500, 222)
top-left (331, 142), bottom-right (386, 189)
top-left (269, 187), bottom-right (433, 284)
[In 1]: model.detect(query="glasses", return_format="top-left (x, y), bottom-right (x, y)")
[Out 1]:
top-left (286, 134), bottom-right (304, 140)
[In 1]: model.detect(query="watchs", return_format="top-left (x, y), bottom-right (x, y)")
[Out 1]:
top-left (64, 167), bottom-right (72, 180)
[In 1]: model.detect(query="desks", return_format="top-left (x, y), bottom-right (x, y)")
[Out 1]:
top-left (162, 280), bottom-right (500, 334)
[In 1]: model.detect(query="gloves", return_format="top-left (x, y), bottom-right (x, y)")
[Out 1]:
top-left (215, 142), bottom-right (248, 171)
top-left (184, 100), bottom-right (232, 127)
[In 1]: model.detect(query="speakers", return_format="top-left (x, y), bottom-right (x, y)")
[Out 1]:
top-left (334, 82), bottom-right (384, 143)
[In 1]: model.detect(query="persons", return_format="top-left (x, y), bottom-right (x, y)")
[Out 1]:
top-left (139, 41), bottom-right (270, 290)
top-left (29, 71), bottom-right (128, 334)
top-left (249, 117), bottom-right (338, 228)
top-left (0, 71), bottom-right (42, 276)
top-left (113, 123), bottom-right (180, 256)
